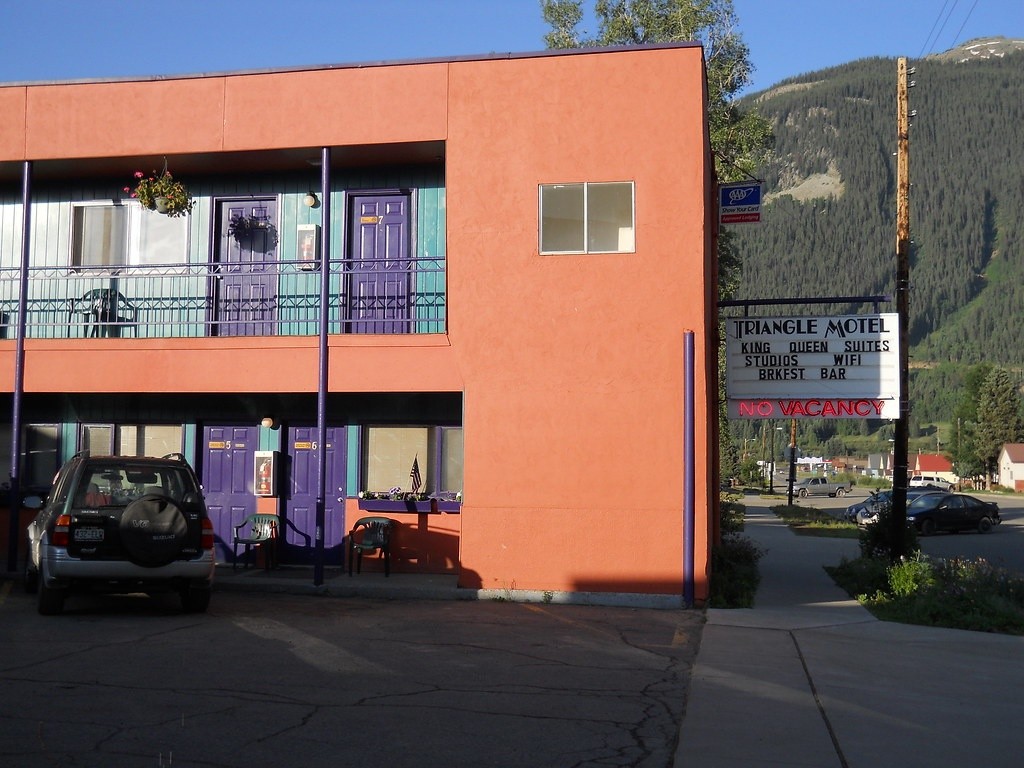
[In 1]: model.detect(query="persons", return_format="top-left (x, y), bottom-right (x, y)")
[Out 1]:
top-left (84, 483), bottom-right (111, 507)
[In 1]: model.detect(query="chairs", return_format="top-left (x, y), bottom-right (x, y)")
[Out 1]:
top-left (233, 514), bottom-right (280, 572)
top-left (143, 486), bottom-right (164, 497)
top-left (69, 288), bottom-right (139, 339)
top-left (85, 482), bottom-right (100, 494)
top-left (349, 517), bottom-right (394, 577)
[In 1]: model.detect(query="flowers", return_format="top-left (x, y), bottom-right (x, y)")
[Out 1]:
top-left (435, 490), bottom-right (461, 502)
top-left (123, 171), bottom-right (196, 218)
top-left (359, 487), bottom-right (433, 501)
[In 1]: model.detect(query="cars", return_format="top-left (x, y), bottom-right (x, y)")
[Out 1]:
top-left (906, 492), bottom-right (1001, 537)
top-left (845, 486), bottom-right (950, 530)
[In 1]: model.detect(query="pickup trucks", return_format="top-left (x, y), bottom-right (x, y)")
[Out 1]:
top-left (784, 476), bottom-right (852, 498)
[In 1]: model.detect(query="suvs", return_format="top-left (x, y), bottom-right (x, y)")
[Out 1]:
top-left (909, 475), bottom-right (955, 494)
top-left (21, 449), bottom-right (216, 617)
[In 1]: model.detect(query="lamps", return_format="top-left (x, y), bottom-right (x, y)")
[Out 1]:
top-left (262, 415), bottom-right (273, 428)
top-left (303, 191), bottom-right (316, 207)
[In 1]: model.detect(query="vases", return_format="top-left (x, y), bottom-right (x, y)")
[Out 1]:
top-left (358, 499), bottom-right (432, 513)
top-left (154, 196), bottom-right (171, 214)
top-left (435, 500), bottom-right (461, 512)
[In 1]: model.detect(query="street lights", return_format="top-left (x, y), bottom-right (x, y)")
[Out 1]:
top-left (744, 438), bottom-right (756, 459)
top-left (761, 427), bottom-right (784, 492)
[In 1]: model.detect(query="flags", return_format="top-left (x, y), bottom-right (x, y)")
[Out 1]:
top-left (411, 459), bottom-right (422, 491)
